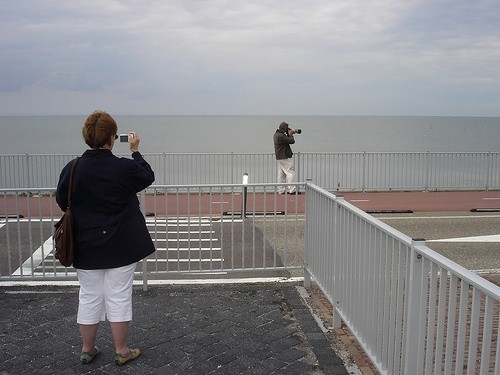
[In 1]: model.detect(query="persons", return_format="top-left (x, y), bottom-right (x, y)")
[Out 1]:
top-left (272, 122), bottom-right (302, 196)
top-left (52, 109), bottom-right (154, 366)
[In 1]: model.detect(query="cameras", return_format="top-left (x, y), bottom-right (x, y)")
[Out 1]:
top-left (286, 128), bottom-right (301, 134)
top-left (119, 134), bottom-right (134, 143)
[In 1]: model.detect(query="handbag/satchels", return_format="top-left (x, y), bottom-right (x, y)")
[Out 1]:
top-left (52, 206), bottom-right (73, 267)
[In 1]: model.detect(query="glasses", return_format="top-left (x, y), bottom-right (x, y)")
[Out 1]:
top-left (115, 135), bottom-right (118, 140)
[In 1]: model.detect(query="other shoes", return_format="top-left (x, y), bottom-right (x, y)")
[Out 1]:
top-left (115, 348), bottom-right (140, 366)
top-left (290, 191), bottom-right (301, 195)
top-left (80, 346), bottom-right (100, 364)
